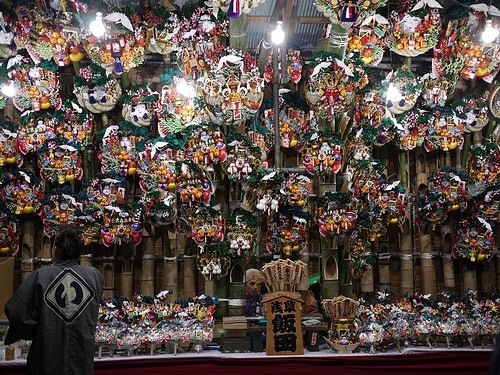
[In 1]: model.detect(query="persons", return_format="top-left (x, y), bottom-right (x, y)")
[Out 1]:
top-left (242, 268), bottom-right (269, 351)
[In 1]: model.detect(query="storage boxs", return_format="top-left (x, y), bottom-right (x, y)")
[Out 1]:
top-left (212, 316), bottom-right (265, 354)
